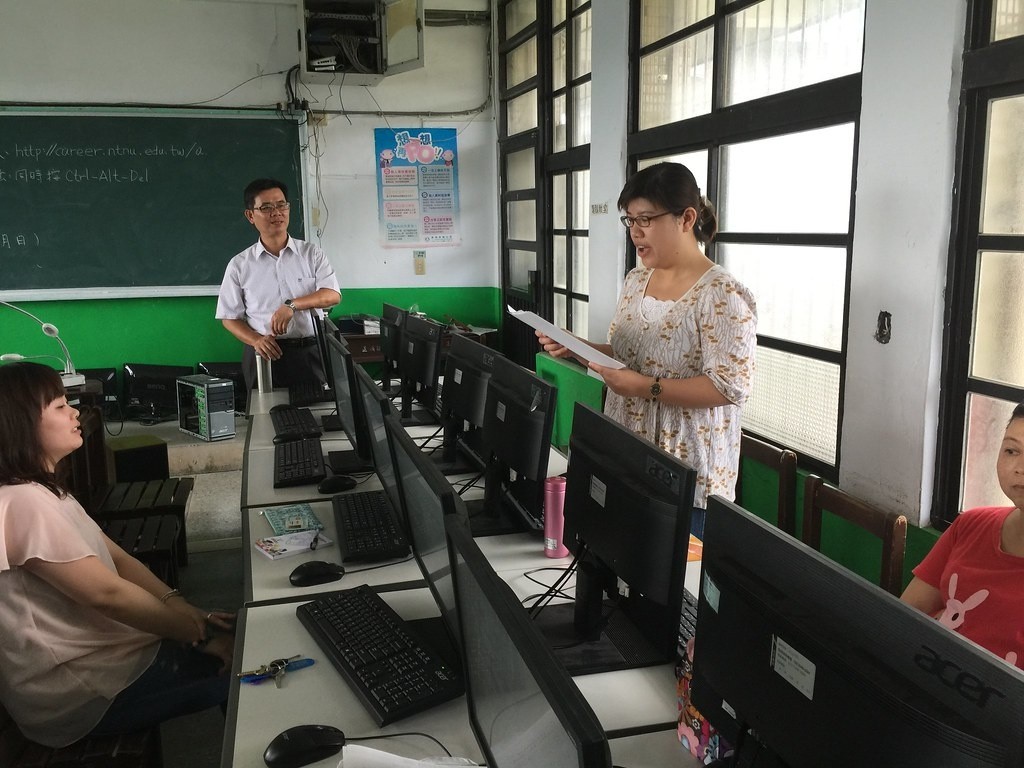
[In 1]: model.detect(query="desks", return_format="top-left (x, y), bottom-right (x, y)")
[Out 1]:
top-left (339, 332), bottom-right (486, 390)
top-left (220, 378), bottom-right (702, 768)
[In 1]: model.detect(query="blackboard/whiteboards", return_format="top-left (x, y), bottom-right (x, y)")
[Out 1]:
top-left (0, 101), bottom-right (318, 303)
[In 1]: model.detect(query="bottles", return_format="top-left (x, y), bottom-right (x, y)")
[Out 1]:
top-left (256, 352), bottom-right (273, 393)
top-left (544, 477), bottom-right (569, 558)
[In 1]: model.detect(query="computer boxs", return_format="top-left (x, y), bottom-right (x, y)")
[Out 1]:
top-left (176, 375), bottom-right (236, 441)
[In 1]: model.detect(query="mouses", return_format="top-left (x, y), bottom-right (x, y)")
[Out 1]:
top-left (264, 724), bottom-right (345, 768)
top-left (318, 474), bottom-right (357, 494)
top-left (288, 561), bottom-right (346, 587)
top-left (272, 433), bottom-right (302, 445)
top-left (269, 403), bottom-right (298, 411)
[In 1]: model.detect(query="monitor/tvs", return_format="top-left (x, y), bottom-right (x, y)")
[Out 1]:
top-left (310, 302), bottom-right (1024, 768)
top-left (196, 360), bottom-right (248, 414)
top-left (121, 362), bottom-right (196, 424)
top-left (54, 368), bottom-right (121, 422)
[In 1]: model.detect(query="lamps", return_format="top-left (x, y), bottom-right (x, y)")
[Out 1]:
top-left (0, 301), bottom-right (85, 387)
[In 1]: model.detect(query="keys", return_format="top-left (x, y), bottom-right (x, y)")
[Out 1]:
top-left (238, 654), bottom-right (301, 688)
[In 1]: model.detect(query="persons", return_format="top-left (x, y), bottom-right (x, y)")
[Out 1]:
top-left (0, 362), bottom-right (236, 768)
top-left (687, 402), bottom-right (1024, 670)
top-left (215, 179), bottom-right (342, 398)
top-left (535, 163), bottom-right (757, 542)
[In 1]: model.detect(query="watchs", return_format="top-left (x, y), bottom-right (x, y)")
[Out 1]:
top-left (650, 377), bottom-right (662, 402)
top-left (284, 299), bottom-right (296, 312)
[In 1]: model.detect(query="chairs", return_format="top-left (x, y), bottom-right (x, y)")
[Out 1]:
top-left (602, 384), bottom-right (907, 599)
top-left (0, 405), bottom-right (194, 768)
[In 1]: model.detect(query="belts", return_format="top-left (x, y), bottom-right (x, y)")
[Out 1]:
top-left (275, 338), bottom-right (316, 349)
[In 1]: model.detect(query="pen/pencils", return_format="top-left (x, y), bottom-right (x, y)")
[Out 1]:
top-left (310, 537), bottom-right (317, 550)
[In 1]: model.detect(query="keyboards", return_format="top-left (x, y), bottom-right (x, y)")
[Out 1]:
top-left (675, 585), bottom-right (698, 658)
top-left (297, 583), bottom-right (467, 728)
top-left (332, 490), bottom-right (412, 564)
top-left (270, 409), bottom-right (322, 437)
top-left (274, 438), bottom-right (328, 489)
top-left (500, 485), bottom-right (545, 536)
top-left (288, 378), bottom-right (324, 406)
top-left (425, 383), bottom-right (444, 425)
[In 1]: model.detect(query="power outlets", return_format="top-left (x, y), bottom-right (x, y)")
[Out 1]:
top-left (307, 113), bottom-right (328, 126)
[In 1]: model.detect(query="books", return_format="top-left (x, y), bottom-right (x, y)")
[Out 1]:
top-left (255, 530), bottom-right (333, 560)
top-left (263, 503), bottom-right (324, 535)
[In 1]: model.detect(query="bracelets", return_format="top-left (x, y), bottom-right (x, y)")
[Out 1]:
top-left (160, 589), bottom-right (180, 603)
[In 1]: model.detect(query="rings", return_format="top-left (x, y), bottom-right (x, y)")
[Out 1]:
top-left (207, 614), bottom-right (211, 619)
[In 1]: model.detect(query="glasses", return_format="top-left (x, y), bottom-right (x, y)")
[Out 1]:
top-left (252, 201), bottom-right (290, 213)
top-left (620, 206), bottom-right (685, 228)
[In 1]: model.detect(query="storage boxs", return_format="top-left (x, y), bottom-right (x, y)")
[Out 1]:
top-left (107, 435), bottom-right (169, 483)
